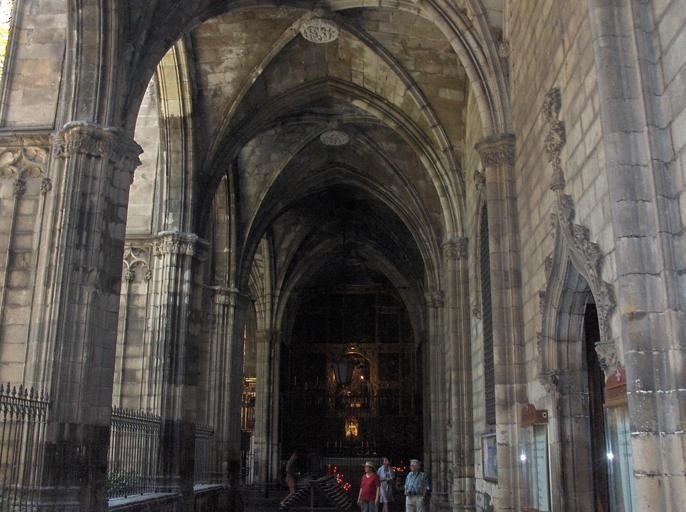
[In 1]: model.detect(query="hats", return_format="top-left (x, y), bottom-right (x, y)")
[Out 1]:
top-left (363, 461), bottom-right (375, 471)
top-left (410, 460), bottom-right (421, 464)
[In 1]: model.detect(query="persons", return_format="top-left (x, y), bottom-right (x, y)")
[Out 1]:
top-left (404, 459), bottom-right (429, 512)
top-left (285, 450), bottom-right (300, 493)
top-left (356, 460), bottom-right (381, 512)
top-left (377, 457), bottom-right (395, 512)
